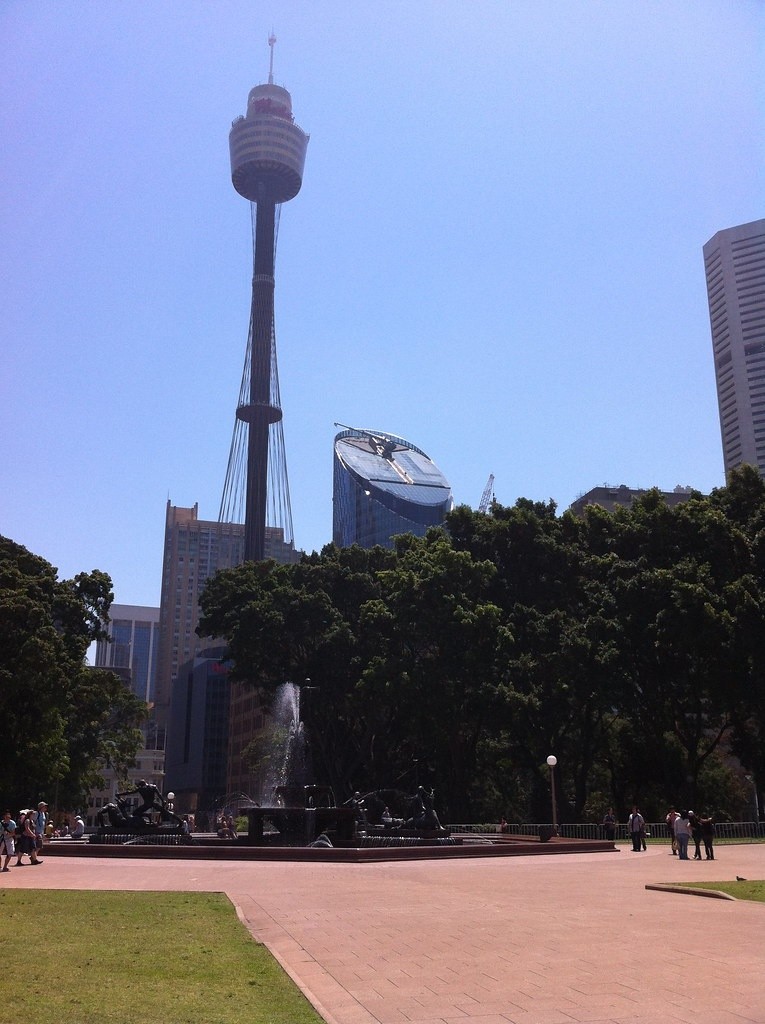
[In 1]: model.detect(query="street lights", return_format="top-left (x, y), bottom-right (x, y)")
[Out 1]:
top-left (167, 792), bottom-right (174, 812)
top-left (548, 755), bottom-right (558, 834)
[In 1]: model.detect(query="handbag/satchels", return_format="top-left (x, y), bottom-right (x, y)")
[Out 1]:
top-left (672, 839), bottom-right (680, 850)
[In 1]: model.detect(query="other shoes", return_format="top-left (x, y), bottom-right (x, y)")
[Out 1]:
top-left (706, 856), bottom-right (714, 860)
top-left (31, 859), bottom-right (43, 865)
top-left (631, 848), bottom-right (641, 852)
top-left (694, 855), bottom-right (702, 860)
top-left (0, 866), bottom-right (11, 872)
top-left (681, 857), bottom-right (690, 859)
top-left (17, 861), bottom-right (24, 865)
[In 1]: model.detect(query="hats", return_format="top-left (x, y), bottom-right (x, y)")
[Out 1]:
top-left (38, 802), bottom-right (48, 809)
top-left (688, 811), bottom-right (694, 816)
top-left (74, 816), bottom-right (81, 819)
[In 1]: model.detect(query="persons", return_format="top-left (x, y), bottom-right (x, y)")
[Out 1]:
top-left (189, 816), bottom-right (195, 833)
top-left (666, 805), bottom-right (682, 855)
top-left (115, 779), bottom-right (184, 828)
top-left (31, 801), bottom-right (49, 858)
top-left (352, 791), bottom-right (368, 818)
top-left (46, 819), bottom-right (54, 840)
top-left (70, 815), bottom-right (85, 839)
top-left (95, 797), bottom-right (146, 829)
top-left (0, 810), bottom-right (17, 872)
top-left (222, 816), bottom-right (231, 840)
top-left (603, 808), bottom-right (617, 840)
top-left (228, 816), bottom-right (238, 840)
top-left (628, 808), bottom-right (644, 851)
top-left (381, 807), bottom-right (391, 818)
top-left (16, 810), bottom-right (45, 867)
top-left (635, 809), bottom-right (647, 851)
top-left (686, 810), bottom-right (713, 860)
top-left (700, 813), bottom-right (714, 860)
top-left (499, 816), bottom-right (508, 833)
top-left (404, 786), bottom-right (445, 830)
top-left (674, 809), bottom-right (691, 860)
